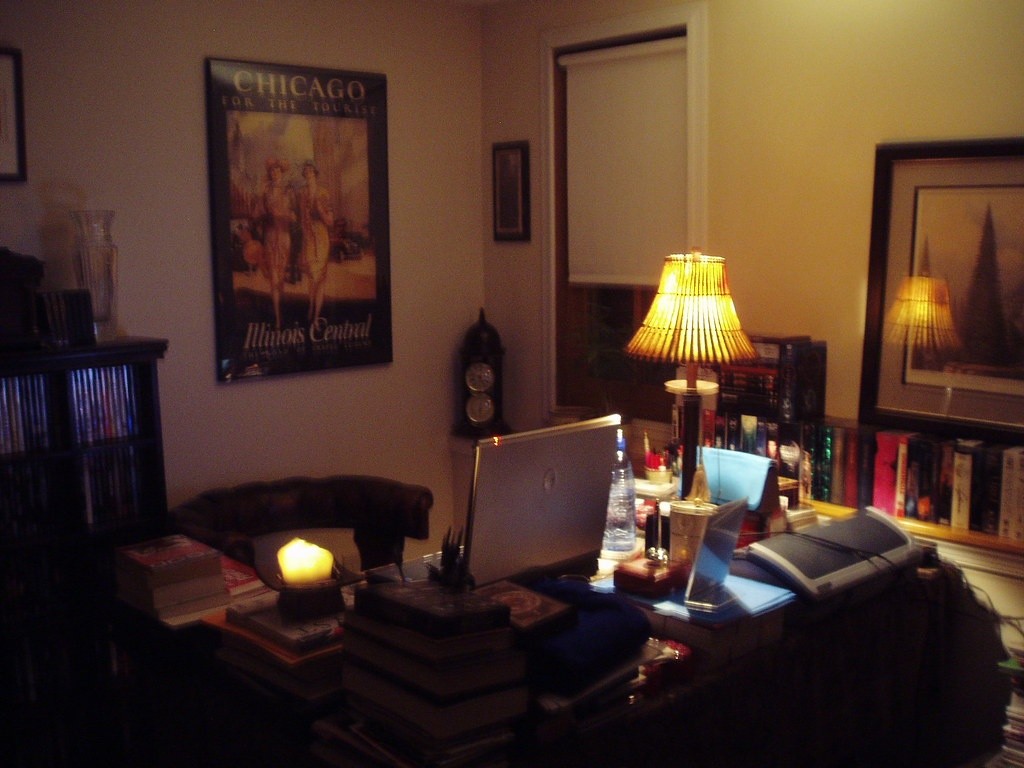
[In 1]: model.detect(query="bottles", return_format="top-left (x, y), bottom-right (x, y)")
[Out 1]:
top-left (602, 438), bottom-right (636, 550)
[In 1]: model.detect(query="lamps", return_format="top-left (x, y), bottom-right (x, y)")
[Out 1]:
top-left (623, 250), bottom-right (753, 500)
top-left (889, 276), bottom-right (967, 350)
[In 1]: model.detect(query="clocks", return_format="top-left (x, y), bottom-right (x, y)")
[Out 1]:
top-left (445, 309), bottom-right (511, 443)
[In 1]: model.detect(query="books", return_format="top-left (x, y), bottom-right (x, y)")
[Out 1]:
top-left (998, 642), bottom-right (1024, 768)
top-left (869, 424), bottom-right (1024, 542)
top-left (0, 365), bottom-right (141, 537)
top-left (112, 534), bottom-right (266, 619)
top-left (672, 341), bottom-right (858, 509)
top-left (199, 580), bottom-right (639, 768)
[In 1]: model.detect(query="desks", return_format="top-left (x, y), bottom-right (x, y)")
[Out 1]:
top-left (109, 557), bottom-right (947, 768)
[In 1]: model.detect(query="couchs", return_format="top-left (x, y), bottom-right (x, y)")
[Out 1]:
top-left (169, 474), bottom-right (434, 587)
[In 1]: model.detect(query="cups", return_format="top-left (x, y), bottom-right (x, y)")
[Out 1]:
top-left (669, 501), bottom-right (719, 582)
top-left (644, 466), bottom-right (673, 483)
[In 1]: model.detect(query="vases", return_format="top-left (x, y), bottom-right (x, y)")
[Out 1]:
top-left (69, 207), bottom-right (120, 341)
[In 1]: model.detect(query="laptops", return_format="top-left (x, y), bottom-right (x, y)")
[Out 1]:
top-left (367, 421), bottom-right (621, 589)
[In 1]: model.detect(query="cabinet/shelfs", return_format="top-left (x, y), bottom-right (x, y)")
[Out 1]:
top-left (809, 499), bottom-right (1024, 722)
top-left (0, 338), bottom-right (169, 658)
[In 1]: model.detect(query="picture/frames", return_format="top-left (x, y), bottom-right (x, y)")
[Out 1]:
top-left (0, 47), bottom-right (27, 182)
top-left (852, 136), bottom-right (1024, 446)
top-left (207, 55), bottom-right (395, 385)
top-left (490, 140), bottom-right (534, 243)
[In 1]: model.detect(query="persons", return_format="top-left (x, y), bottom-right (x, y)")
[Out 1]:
top-left (262, 156), bottom-right (334, 332)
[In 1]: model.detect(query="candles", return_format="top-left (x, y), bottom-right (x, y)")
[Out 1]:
top-left (274, 535), bottom-right (345, 588)
